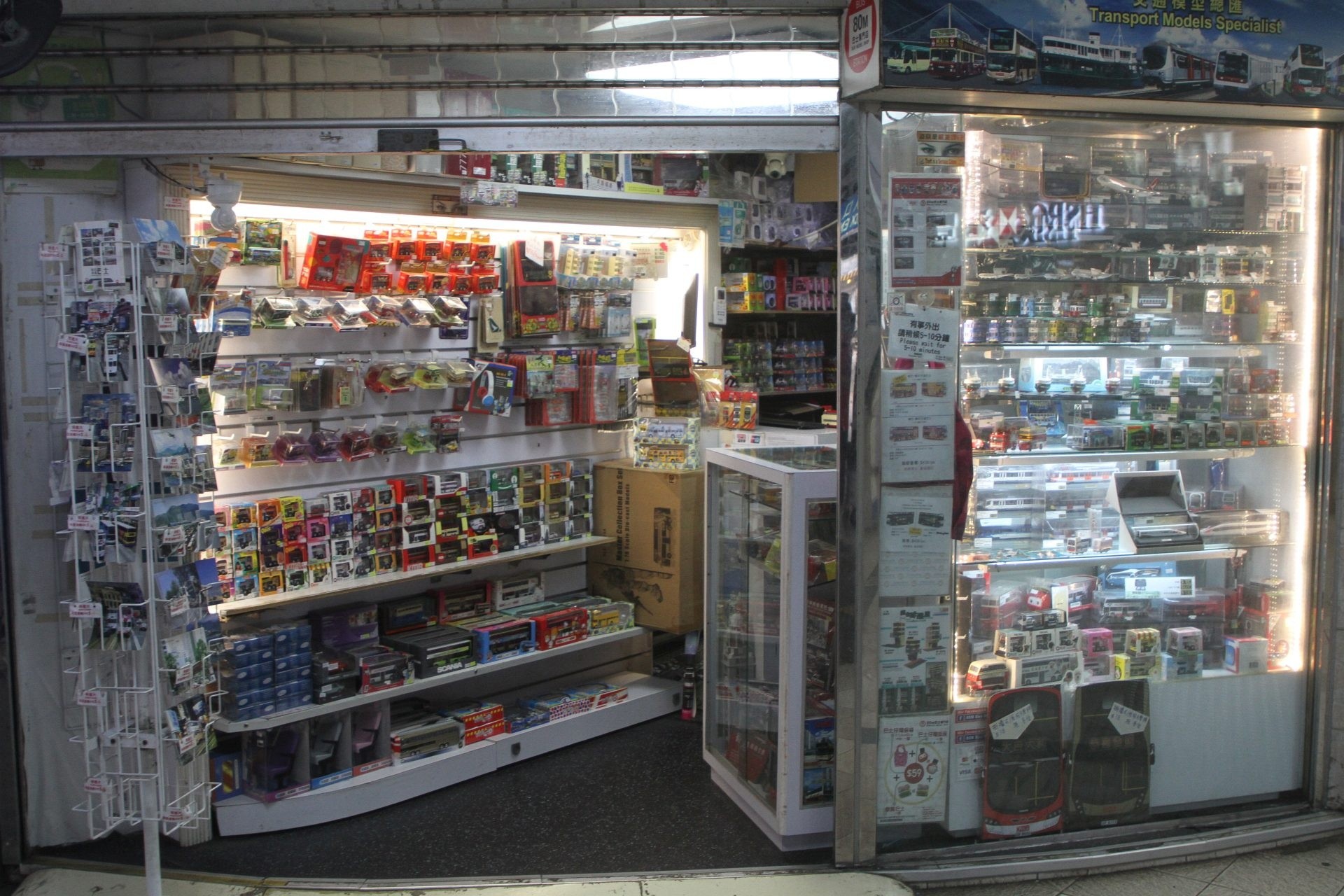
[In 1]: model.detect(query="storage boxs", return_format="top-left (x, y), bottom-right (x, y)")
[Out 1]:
top-left (961, 128), bottom-right (1306, 687)
top-left (717, 171), bottom-right (836, 387)
top-left (143, 27), bottom-right (705, 807)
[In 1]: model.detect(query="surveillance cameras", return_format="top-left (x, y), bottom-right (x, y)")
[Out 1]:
top-left (764, 153), bottom-right (787, 179)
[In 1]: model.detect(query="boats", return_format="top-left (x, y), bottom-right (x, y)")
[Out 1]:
top-left (1039, 4), bottom-right (1143, 89)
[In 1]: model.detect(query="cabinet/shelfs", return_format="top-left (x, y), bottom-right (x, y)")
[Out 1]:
top-left (206, 537), bottom-right (688, 838)
top-left (958, 223), bottom-right (1307, 561)
top-left (702, 443), bottom-right (925, 853)
top-left (728, 241), bottom-right (836, 397)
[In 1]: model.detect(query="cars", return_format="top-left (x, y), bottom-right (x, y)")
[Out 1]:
top-left (976, 685), bottom-right (1071, 845)
top-left (724, 272), bottom-right (838, 391)
top-left (961, 284), bottom-right (1284, 692)
top-left (1064, 678), bottom-right (1155, 831)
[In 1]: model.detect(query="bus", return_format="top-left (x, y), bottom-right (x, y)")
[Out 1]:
top-left (985, 26), bottom-right (1039, 85)
top-left (1283, 43), bottom-right (1327, 102)
top-left (309, 570), bottom-right (636, 706)
top-left (1326, 54), bottom-right (1344, 101)
top-left (1141, 41), bottom-right (1216, 93)
top-left (887, 42), bottom-right (930, 75)
top-left (1213, 48), bottom-right (1286, 100)
top-left (929, 27), bottom-right (987, 80)
